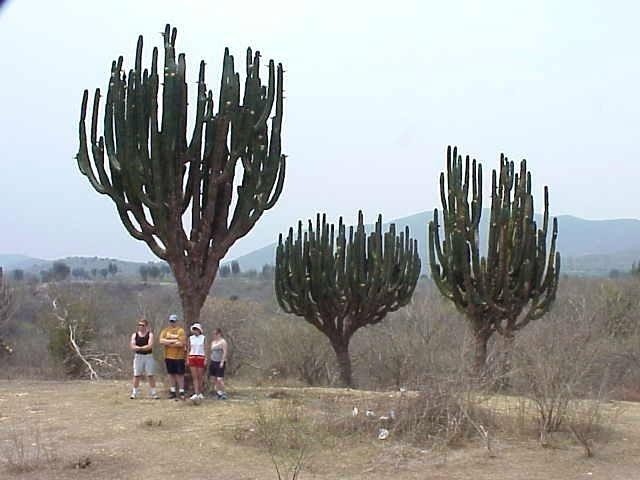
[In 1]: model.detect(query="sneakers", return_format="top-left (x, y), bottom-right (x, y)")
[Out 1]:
top-left (167, 390), bottom-right (186, 400)
top-left (130, 392), bottom-right (137, 399)
top-left (216, 392), bottom-right (227, 400)
top-left (151, 392), bottom-right (160, 399)
top-left (189, 393), bottom-right (203, 400)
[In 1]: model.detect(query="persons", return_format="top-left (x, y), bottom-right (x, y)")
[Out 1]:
top-left (158, 314), bottom-right (228, 405)
top-left (129, 318), bottom-right (160, 399)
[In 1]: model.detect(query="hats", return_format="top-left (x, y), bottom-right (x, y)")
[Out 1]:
top-left (190, 323), bottom-right (203, 333)
top-left (169, 315), bottom-right (177, 321)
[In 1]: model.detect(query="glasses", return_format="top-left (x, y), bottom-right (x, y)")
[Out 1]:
top-left (138, 324), bottom-right (144, 326)
